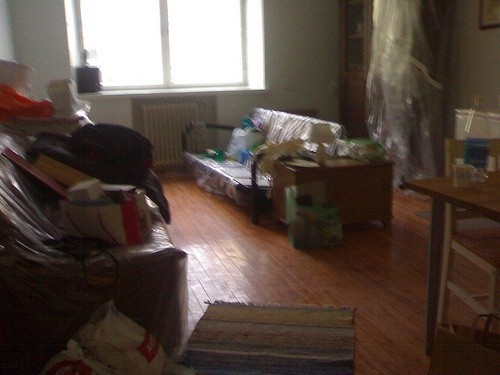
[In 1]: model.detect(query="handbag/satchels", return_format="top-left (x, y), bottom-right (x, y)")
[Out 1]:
top-left (60, 189), bottom-right (151, 248)
top-left (40, 300), bottom-right (167, 375)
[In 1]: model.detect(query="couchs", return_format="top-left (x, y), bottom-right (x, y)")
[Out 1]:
top-left (0, 124), bottom-right (188, 358)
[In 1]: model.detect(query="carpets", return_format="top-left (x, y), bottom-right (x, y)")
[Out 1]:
top-left (174, 299), bottom-right (356, 375)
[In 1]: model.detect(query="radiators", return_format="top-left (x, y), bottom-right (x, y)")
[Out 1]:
top-left (131, 96), bottom-right (218, 169)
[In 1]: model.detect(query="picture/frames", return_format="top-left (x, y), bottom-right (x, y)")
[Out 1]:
top-left (478, 0), bottom-right (500, 29)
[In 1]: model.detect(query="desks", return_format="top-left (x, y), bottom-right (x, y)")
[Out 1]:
top-left (271, 157), bottom-right (394, 251)
top-left (402, 177), bottom-right (500, 357)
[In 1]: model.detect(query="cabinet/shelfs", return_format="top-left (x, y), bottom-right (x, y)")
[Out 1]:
top-left (338, 0), bottom-right (372, 139)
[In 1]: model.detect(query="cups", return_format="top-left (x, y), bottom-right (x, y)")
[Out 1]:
top-left (453, 163), bottom-right (474, 190)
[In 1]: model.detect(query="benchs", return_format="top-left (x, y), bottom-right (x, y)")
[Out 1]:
top-left (180, 108), bottom-right (342, 227)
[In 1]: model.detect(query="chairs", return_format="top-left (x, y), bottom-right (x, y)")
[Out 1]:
top-left (443, 139), bottom-right (500, 183)
